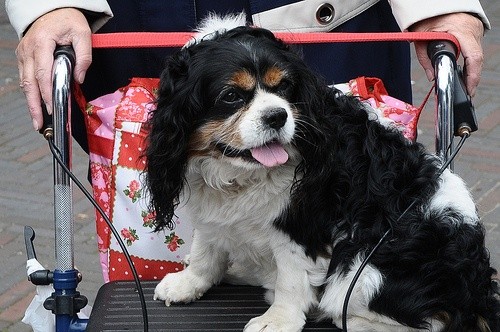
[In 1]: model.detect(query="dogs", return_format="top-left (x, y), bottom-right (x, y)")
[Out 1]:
top-left (135, 8), bottom-right (500, 332)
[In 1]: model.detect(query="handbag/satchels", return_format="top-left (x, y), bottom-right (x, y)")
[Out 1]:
top-left (71, 31), bottom-right (462, 283)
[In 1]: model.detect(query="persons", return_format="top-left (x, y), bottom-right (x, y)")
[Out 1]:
top-left (2, 0), bottom-right (493, 190)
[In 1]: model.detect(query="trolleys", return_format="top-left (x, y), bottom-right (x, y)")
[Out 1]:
top-left (20, 32), bottom-right (480, 331)
